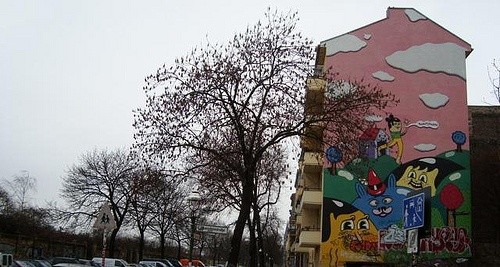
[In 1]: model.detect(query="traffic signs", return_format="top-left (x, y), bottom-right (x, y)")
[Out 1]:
top-left (197, 224), bottom-right (228, 234)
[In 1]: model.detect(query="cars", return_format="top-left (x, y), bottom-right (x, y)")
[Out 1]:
top-left (0, 252), bottom-right (209, 267)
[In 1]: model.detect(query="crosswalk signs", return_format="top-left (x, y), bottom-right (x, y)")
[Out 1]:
top-left (92, 202), bottom-right (117, 229)
top-left (402, 192), bottom-right (425, 231)
